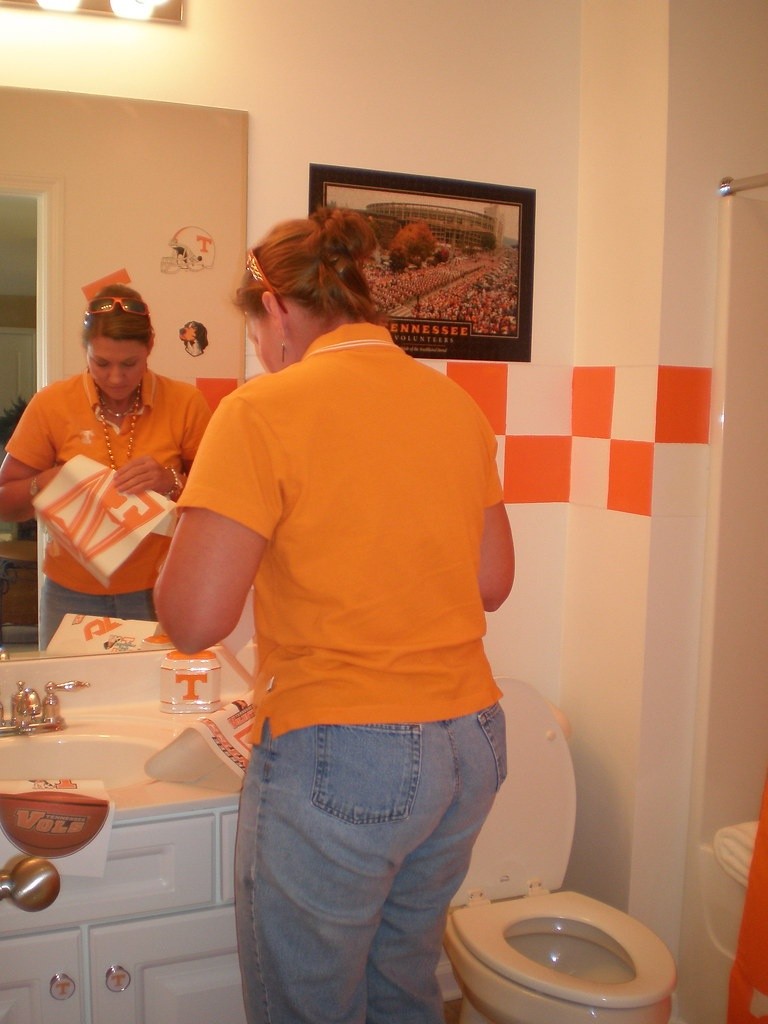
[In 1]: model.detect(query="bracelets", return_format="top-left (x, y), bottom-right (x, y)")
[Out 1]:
top-left (30, 475), bottom-right (41, 498)
top-left (159, 465), bottom-right (179, 498)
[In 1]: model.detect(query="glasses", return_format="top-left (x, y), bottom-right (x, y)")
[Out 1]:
top-left (246, 249), bottom-right (289, 315)
top-left (83, 297), bottom-right (154, 332)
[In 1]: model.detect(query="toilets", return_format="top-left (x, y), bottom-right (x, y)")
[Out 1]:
top-left (444, 676), bottom-right (677, 1024)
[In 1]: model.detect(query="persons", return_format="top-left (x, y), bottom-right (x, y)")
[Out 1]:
top-left (351, 241), bottom-right (521, 338)
top-left (0, 286), bottom-right (216, 658)
top-left (149, 212), bottom-right (515, 1023)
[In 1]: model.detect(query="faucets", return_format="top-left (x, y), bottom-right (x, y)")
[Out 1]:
top-left (10, 681), bottom-right (41, 726)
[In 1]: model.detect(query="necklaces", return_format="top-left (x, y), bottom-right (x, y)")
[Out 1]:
top-left (93, 389), bottom-right (137, 417)
top-left (95, 375), bottom-right (142, 473)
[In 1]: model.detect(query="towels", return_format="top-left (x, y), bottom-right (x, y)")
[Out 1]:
top-left (713, 821), bottom-right (759, 888)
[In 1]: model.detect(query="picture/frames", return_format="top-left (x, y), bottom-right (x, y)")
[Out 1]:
top-left (309, 162), bottom-right (537, 364)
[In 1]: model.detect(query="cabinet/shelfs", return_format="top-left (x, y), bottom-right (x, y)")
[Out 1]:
top-left (0, 806), bottom-right (246, 1024)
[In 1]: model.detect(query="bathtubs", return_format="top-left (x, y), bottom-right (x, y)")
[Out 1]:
top-left (686, 837), bottom-right (745, 1024)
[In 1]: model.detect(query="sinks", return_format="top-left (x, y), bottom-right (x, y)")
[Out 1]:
top-left (0, 715), bottom-right (187, 789)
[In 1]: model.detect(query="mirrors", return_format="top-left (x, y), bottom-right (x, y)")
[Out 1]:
top-left (0, 84), bottom-right (251, 663)
